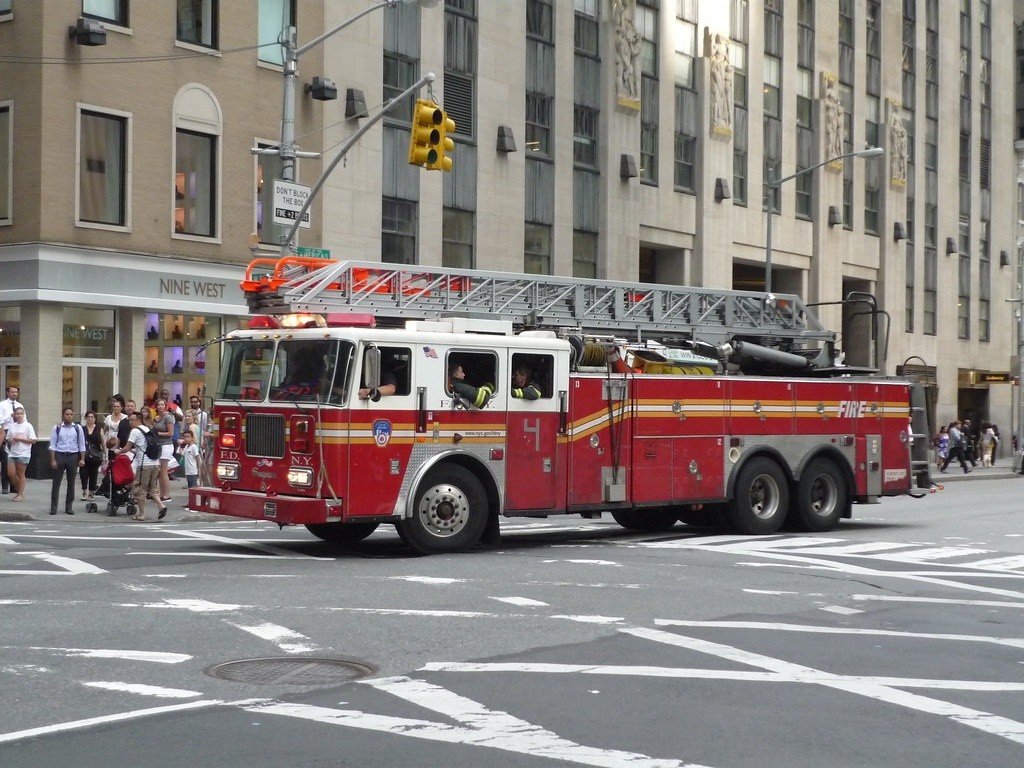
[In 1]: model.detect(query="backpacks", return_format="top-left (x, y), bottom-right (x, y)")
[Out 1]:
top-left (134, 425), bottom-right (163, 460)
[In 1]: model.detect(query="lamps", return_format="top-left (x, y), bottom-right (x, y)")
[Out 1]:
top-left (347, 87), bottom-right (369, 118)
top-left (496, 126), bottom-right (517, 152)
top-left (894, 222), bottom-right (907, 241)
top-left (946, 237), bottom-right (959, 254)
top-left (304, 75), bottom-right (338, 100)
top-left (829, 206), bottom-right (841, 226)
top-left (1000, 250), bottom-right (1011, 266)
top-left (620, 154), bottom-right (638, 179)
top-left (67, 18), bottom-right (107, 48)
top-left (716, 177), bottom-right (730, 200)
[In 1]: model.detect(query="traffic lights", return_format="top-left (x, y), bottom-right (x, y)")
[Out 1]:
top-left (426, 111), bottom-right (456, 172)
top-left (409, 98), bottom-right (445, 166)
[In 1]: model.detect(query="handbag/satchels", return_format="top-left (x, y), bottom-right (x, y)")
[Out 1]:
top-left (112, 455), bottom-right (135, 484)
top-left (84, 442), bottom-right (104, 467)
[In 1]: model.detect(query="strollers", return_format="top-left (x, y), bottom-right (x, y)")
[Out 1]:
top-left (86, 448), bottom-right (140, 516)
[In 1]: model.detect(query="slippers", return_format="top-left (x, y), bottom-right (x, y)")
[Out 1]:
top-left (132, 515), bottom-right (145, 522)
top-left (157, 506), bottom-right (168, 520)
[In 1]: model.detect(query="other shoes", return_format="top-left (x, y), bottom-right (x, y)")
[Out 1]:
top-left (182, 485), bottom-right (188, 489)
top-left (12, 494), bottom-right (26, 502)
top-left (65, 509), bottom-right (75, 515)
top-left (81, 492), bottom-right (95, 501)
top-left (50, 509), bottom-right (57, 515)
top-left (1, 488), bottom-right (18, 494)
top-left (160, 496), bottom-right (172, 501)
top-left (965, 469), bottom-right (972, 474)
top-left (973, 464), bottom-right (979, 467)
top-left (940, 470), bottom-right (947, 474)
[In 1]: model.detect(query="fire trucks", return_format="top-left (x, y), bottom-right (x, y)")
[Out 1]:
top-left (185, 259), bottom-right (944, 558)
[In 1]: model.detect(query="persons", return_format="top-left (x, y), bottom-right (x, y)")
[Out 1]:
top-left (0, 382), bottom-right (36, 502)
top-left (48, 390), bottom-right (214, 521)
top-left (174, 359), bottom-right (182, 373)
top-left (908, 417), bottom-right (917, 476)
top-left (932, 419), bottom-right (1001, 474)
top-left (1018, 455), bottom-right (1024, 475)
top-left (175, 221), bottom-right (184, 233)
top-left (279, 346), bottom-right (541, 409)
top-left (150, 360), bottom-right (158, 373)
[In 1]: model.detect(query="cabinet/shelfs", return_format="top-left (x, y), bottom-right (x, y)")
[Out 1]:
top-left (144, 313), bottom-right (219, 412)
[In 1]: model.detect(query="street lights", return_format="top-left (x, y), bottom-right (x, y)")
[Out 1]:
top-left (280, 1), bottom-right (442, 258)
top-left (765, 147), bottom-right (885, 293)
